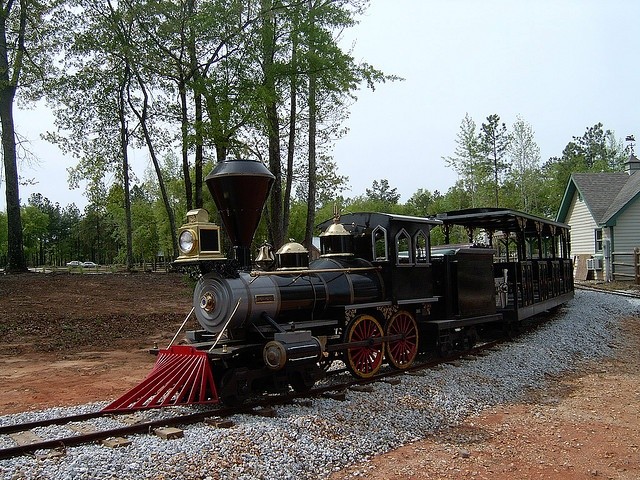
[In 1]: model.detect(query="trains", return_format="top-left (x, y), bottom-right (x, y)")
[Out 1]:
top-left (104, 160), bottom-right (576, 411)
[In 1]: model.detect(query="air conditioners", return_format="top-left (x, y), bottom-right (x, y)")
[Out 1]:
top-left (586, 258), bottom-right (600, 270)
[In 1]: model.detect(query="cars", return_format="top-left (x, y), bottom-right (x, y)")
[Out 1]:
top-left (67, 261), bottom-right (83, 268)
top-left (81, 261), bottom-right (102, 268)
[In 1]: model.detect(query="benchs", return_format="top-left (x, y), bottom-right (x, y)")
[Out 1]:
top-left (494, 264), bottom-right (522, 304)
top-left (532, 261), bottom-right (540, 302)
top-left (559, 259), bottom-right (564, 295)
top-left (547, 260), bottom-right (553, 297)
